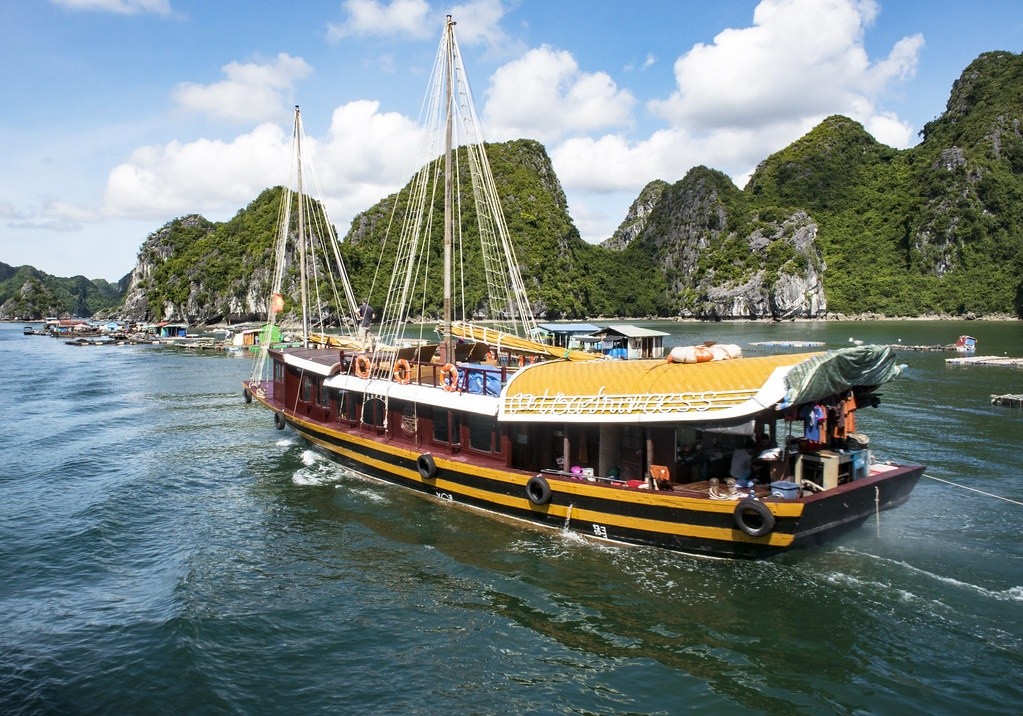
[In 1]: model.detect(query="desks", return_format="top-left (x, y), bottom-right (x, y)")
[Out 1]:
top-left (794, 452), bottom-right (850, 493)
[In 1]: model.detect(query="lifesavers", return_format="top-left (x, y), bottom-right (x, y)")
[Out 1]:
top-left (273, 410), bottom-right (286, 431)
top-left (416, 454), bottom-right (437, 479)
top-left (734, 498), bottom-right (776, 538)
top-left (525, 474), bottom-right (554, 505)
top-left (438, 362), bottom-right (459, 393)
top-left (242, 387), bottom-right (253, 404)
top-left (392, 359), bottom-right (411, 386)
top-left (354, 355), bottom-right (372, 380)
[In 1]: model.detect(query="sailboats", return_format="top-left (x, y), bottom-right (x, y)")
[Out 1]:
top-left (241, 12), bottom-right (930, 562)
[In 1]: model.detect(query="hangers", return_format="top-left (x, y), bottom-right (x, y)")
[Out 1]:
top-left (808, 388), bottom-right (856, 406)
